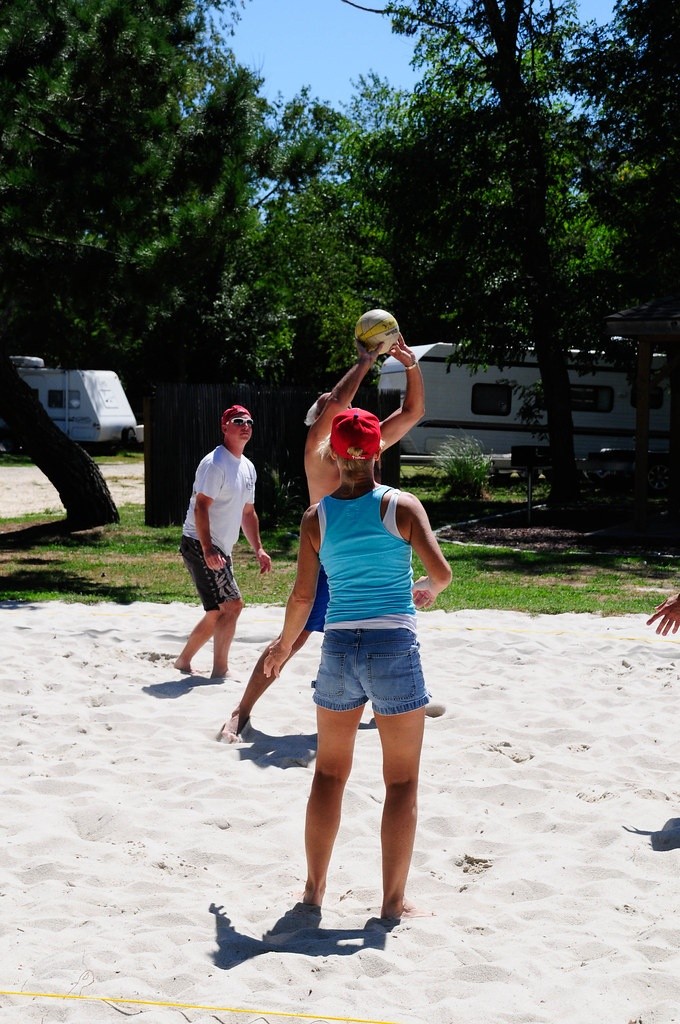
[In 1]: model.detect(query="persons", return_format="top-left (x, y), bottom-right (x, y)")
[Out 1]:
top-left (228, 333), bottom-right (427, 730)
top-left (175, 403), bottom-right (271, 673)
top-left (261, 407), bottom-right (453, 919)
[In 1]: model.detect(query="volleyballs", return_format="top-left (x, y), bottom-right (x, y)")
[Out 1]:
top-left (354, 308), bottom-right (401, 355)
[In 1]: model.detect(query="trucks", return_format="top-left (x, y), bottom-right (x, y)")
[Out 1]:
top-left (378, 342), bottom-right (672, 495)
top-left (0, 356), bottom-right (138, 455)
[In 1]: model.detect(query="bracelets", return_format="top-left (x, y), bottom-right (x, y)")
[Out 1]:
top-left (405, 362), bottom-right (418, 370)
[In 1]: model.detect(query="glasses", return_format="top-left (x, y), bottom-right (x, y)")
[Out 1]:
top-left (227, 418), bottom-right (253, 426)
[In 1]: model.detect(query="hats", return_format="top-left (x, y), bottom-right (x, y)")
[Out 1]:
top-left (329, 408), bottom-right (381, 460)
top-left (221, 405), bottom-right (251, 424)
